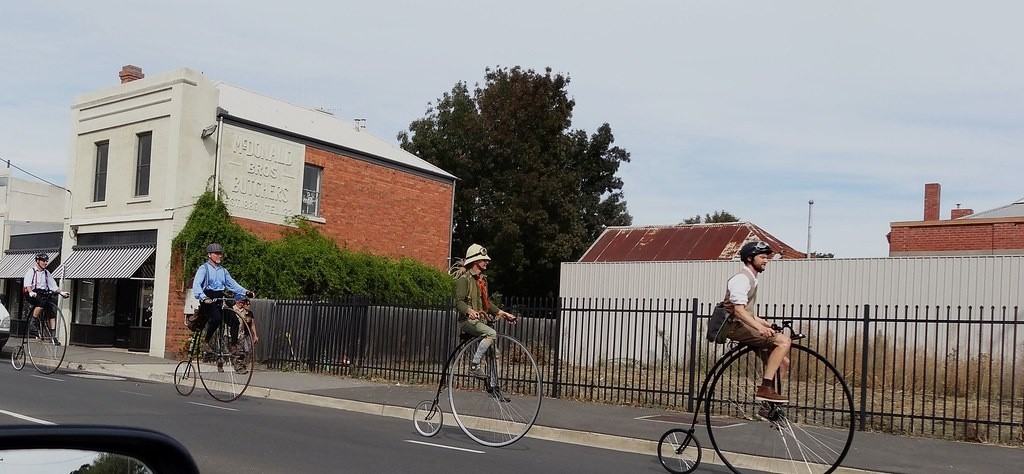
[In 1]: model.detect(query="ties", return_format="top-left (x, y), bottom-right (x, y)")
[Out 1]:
top-left (474, 276), bottom-right (490, 309)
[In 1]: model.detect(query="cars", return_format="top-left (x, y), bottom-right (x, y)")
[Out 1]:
top-left (0, 299), bottom-right (11, 354)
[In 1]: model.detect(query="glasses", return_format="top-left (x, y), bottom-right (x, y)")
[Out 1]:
top-left (755, 242), bottom-right (769, 249)
top-left (246, 303), bottom-right (251, 306)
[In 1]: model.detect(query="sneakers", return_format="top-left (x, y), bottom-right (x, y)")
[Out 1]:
top-left (756, 408), bottom-right (790, 430)
top-left (755, 385), bottom-right (789, 403)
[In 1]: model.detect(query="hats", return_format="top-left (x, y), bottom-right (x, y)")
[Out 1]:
top-left (207, 243), bottom-right (223, 254)
top-left (463, 243), bottom-right (491, 267)
top-left (234, 294), bottom-right (246, 303)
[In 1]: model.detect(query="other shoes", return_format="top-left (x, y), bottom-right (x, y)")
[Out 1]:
top-left (218, 366), bottom-right (224, 372)
top-left (235, 368), bottom-right (246, 373)
top-left (29, 317), bottom-right (40, 337)
top-left (468, 363), bottom-right (485, 377)
top-left (50, 337), bottom-right (62, 346)
top-left (488, 388), bottom-right (511, 401)
top-left (203, 341), bottom-right (212, 353)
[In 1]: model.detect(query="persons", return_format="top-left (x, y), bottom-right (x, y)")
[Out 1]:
top-left (193, 244), bottom-right (255, 357)
top-left (455, 243), bottom-right (518, 402)
top-left (217, 299), bottom-right (260, 373)
top-left (24, 254), bottom-right (71, 345)
top-left (722, 241), bottom-right (791, 431)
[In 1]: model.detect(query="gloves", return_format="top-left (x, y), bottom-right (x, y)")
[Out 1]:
top-left (60, 292), bottom-right (70, 298)
top-left (29, 291), bottom-right (37, 298)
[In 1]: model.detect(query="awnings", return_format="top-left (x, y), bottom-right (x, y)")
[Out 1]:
top-left (0, 252), bottom-right (59, 278)
top-left (51, 246), bottom-right (157, 278)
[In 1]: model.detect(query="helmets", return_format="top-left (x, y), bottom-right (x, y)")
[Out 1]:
top-left (35, 254), bottom-right (48, 262)
top-left (740, 241), bottom-right (773, 262)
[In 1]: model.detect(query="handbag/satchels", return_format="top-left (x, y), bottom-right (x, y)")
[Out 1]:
top-left (707, 305), bottom-right (731, 344)
top-left (187, 310), bottom-right (199, 331)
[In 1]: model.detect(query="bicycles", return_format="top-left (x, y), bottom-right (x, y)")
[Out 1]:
top-left (10, 286), bottom-right (71, 376)
top-left (657, 321), bottom-right (857, 474)
top-left (413, 310), bottom-right (543, 448)
top-left (174, 293), bottom-right (257, 402)
